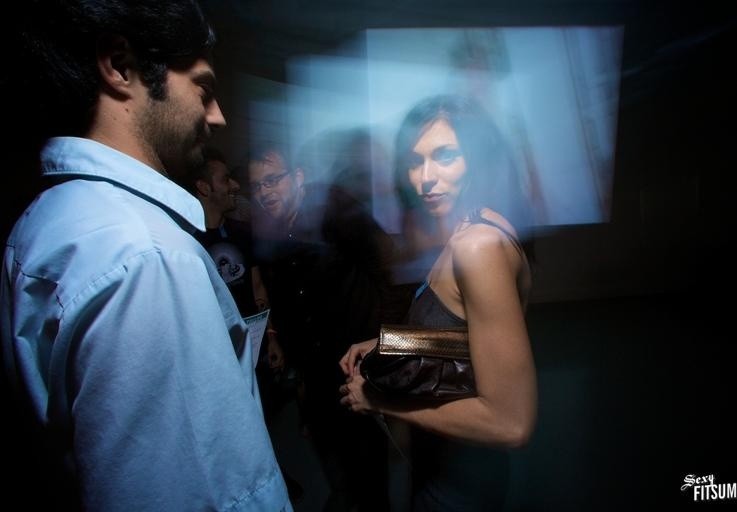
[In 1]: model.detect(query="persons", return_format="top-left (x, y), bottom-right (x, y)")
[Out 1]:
top-left (1, 1), bottom-right (541, 512)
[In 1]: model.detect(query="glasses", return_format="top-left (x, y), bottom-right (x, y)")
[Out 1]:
top-left (249, 170), bottom-right (290, 195)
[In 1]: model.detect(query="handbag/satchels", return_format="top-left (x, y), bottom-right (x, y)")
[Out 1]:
top-left (360, 326), bottom-right (477, 412)
